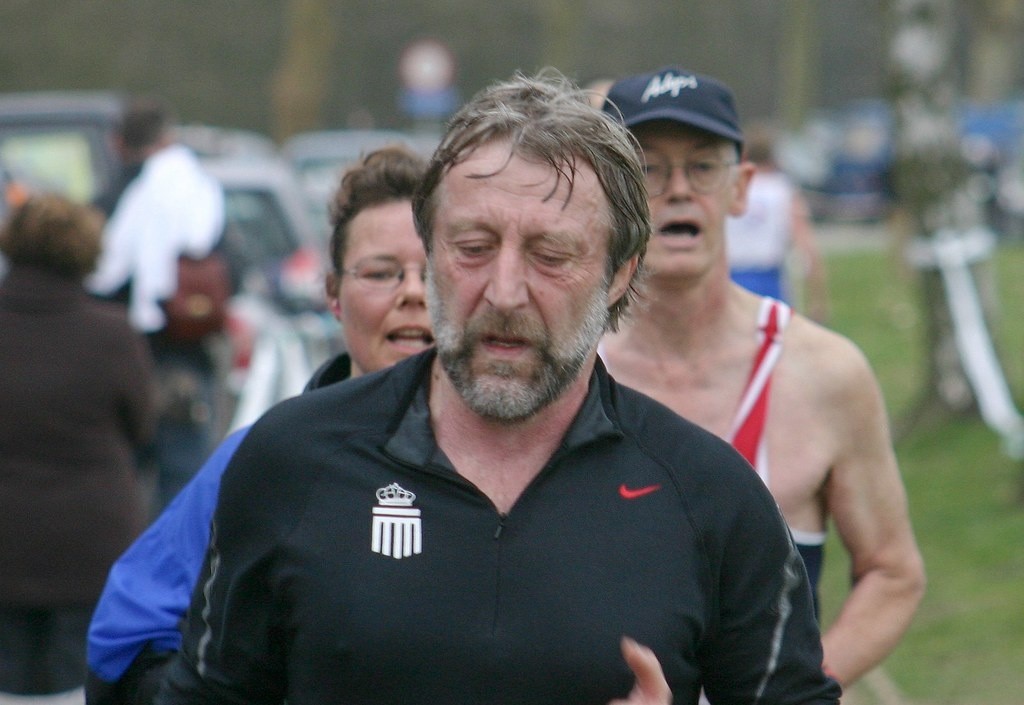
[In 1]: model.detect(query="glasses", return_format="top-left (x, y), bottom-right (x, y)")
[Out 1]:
top-left (339, 253), bottom-right (426, 293)
top-left (644, 147), bottom-right (740, 199)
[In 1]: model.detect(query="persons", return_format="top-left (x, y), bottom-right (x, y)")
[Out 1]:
top-left (729, 136), bottom-right (827, 323)
top-left (0, 94), bottom-right (243, 705)
top-left (599, 71), bottom-right (928, 695)
top-left (85, 145), bottom-right (436, 705)
top-left (162, 64), bottom-right (845, 705)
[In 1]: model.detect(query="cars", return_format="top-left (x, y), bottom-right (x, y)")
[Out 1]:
top-left (0, 85), bottom-right (450, 415)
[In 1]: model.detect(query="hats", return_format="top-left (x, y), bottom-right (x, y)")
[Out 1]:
top-left (605, 66), bottom-right (746, 143)
